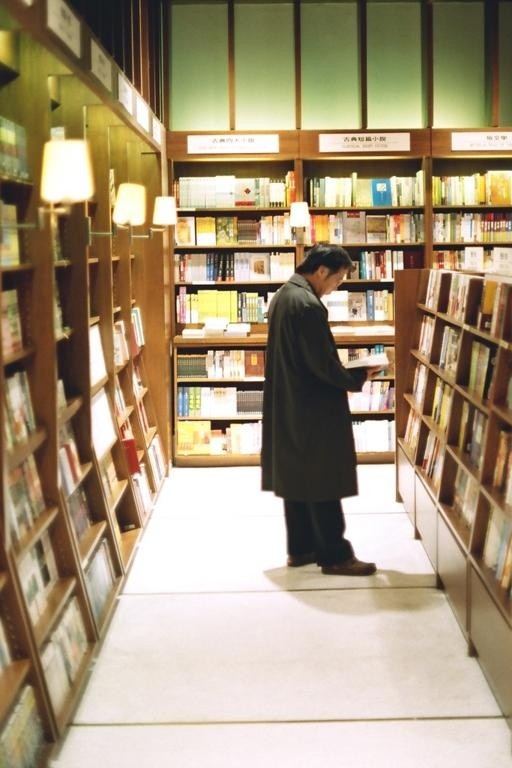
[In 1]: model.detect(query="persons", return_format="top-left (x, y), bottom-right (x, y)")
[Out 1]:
top-left (255, 242), bottom-right (380, 580)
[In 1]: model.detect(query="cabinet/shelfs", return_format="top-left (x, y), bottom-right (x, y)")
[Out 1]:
top-left (165, 127), bottom-right (512, 467)
top-left (0, 1), bottom-right (166, 768)
top-left (289, 201), bottom-right (311, 246)
top-left (395, 269), bottom-right (512, 733)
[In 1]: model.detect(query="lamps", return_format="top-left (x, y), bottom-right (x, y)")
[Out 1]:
top-left (37, 138), bottom-right (179, 247)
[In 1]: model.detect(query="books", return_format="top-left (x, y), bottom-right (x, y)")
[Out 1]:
top-left (174, 169), bottom-right (296, 455)
top-left (85, 306), bottom-right (169, 622)
top-left (415, 465), bottom-right (512, 613)
top-left (337, 346), bottom-right (395, 453)
top-left (403, 271), bottom-right (512, 488)
top-left (304, 168), bottom-right (424, 244)
top-left (432, 170), bottom-right (511, 270)
top-left (2, 112), bottom-right (88, 765)
top-left (321, 249), bottom-right (424, 321)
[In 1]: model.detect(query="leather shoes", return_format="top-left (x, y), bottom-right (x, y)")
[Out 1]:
top-left (287, 551), bottom-right (318, 566)
top-left (322, 555), bottom-right (376, 575)
